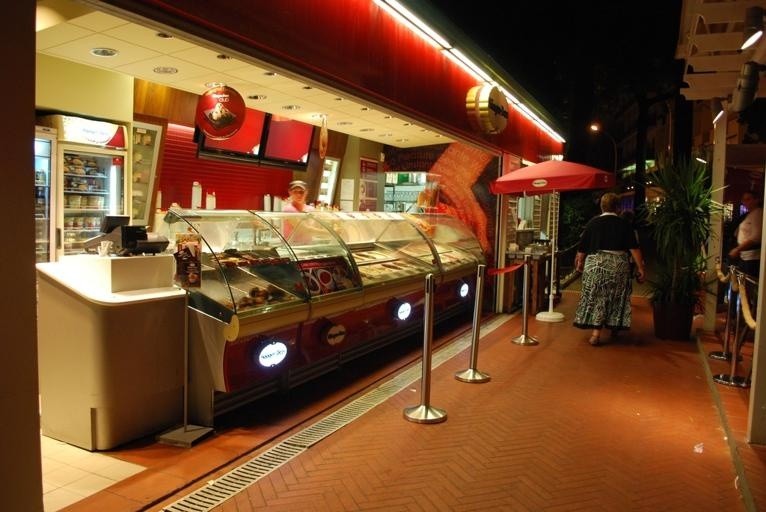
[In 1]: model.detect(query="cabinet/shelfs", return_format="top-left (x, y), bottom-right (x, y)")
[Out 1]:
top-left (33, 115), bottom-right (167, 267)
top-left (163, 207), bottom-right (490, 433)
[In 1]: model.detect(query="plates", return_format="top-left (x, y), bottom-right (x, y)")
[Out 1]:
top-left (301, 269), bottom-right (333, 293)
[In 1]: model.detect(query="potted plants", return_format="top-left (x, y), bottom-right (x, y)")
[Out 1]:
top-left (634, 145), bottom-right (728, 340)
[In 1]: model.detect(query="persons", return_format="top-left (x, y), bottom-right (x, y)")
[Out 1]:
top-left (622, 208), bottom-right (640, 278)
top-left (727, 188), bottom-right (763, 344)
top-left (279, 179), bottom-right (343, 246)
top-left (574, 192), bottom-right (647, 346)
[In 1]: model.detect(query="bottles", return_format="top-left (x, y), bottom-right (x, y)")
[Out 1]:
top-left (416, 172), bottom-right (441, 208)
top-left (508, 243), bottom-right (536, 259)
top-left (153, 187), bottom-right (162, 214)
top-left (191, 182), bottom-right (216, 210)
top-left (263, 193), bottom-right (282, 211)
top-left (309, 200), bottom-right (339, 212)
top-left (35, 169), bottom-right (46, 217)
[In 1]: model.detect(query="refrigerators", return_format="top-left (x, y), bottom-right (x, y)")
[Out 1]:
top-left (34, 125), bottom-right (58, 267)
top-left (38, 113), bottom-right (129, 263)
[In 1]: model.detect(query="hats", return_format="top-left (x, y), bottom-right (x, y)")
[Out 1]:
top-left (287, 179), bottom-right (308, 192)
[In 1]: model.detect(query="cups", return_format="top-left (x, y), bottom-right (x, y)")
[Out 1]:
top-left (65, 195), bottom-right (104, 230)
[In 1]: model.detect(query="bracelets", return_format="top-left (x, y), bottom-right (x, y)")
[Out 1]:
top-left (736, 246), bottom-right (742, 252)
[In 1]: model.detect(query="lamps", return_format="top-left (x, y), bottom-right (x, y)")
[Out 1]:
top-left (739, 6), bottom-right (765, 52)
top-left (694, 142), bottom-right (713, 165)
top-left (709, 93), bottom-right (733, 125)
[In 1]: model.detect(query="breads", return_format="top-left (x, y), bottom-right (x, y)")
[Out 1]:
top-left (210, 249), bottom-right (293, 266)
top-left (221, 285), bottom-right (297, 312)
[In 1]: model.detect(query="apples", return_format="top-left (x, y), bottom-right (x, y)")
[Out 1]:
top-left (212, 103), bottom-right (231, 120)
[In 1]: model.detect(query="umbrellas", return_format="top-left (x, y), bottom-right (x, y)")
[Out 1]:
top-left (491, 157), bottom-right (617, 295)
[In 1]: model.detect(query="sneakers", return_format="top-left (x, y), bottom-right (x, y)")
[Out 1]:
top-left (589, 335), bottom-right (624, 346)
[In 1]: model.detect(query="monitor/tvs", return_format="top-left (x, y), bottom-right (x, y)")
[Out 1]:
top-left (193, 106), bottom-right (316, 172)
